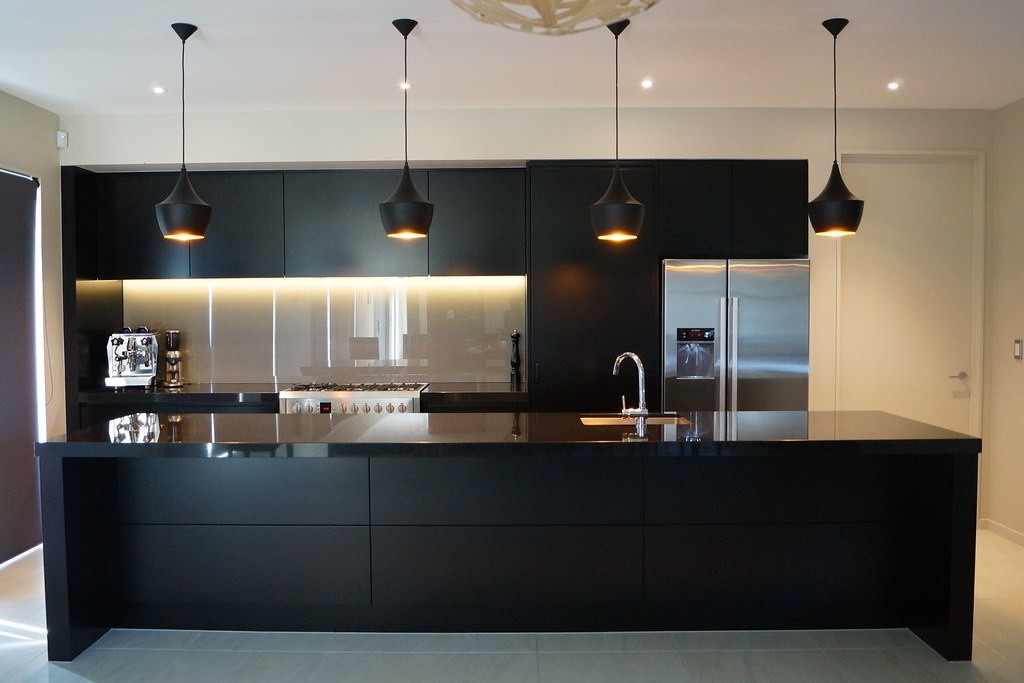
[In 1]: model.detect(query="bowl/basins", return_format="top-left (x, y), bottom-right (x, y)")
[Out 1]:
top-left (120, 327), bottom-right (131, 334)
top-left (135, 326), bottom-right (148, 333)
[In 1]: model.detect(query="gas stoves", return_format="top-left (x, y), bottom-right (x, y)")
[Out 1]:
top-left (279, 382), bottom-right (429, 413)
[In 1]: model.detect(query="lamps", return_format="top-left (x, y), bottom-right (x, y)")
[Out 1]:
top-left (806, 16), bottom-right (865, 237)
top-left (154, 23), bottom-right (213, 239)
top-left (589, 16), bottom-right (643, 241)
top-left (379, 19), bottom-right (434, 238)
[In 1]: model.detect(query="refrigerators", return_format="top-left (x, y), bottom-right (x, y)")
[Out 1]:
top-left (662, 259), bottom-right (810, 411)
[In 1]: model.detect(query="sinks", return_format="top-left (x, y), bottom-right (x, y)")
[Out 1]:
top-left (578, 414), bottom-right (693, 426)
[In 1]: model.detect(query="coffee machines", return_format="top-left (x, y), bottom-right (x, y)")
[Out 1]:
top-left (168, 415), bottom-right (183, 442)
top-left (104, 333), bottom-right (159, 390)
top-left (163, 330), bottom-right (183, 387)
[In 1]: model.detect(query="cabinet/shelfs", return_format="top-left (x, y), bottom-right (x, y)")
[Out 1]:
top-left (61, 159), bottom-right (808, 413)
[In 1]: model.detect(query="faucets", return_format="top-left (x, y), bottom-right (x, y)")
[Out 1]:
top-left (612, 351), bottom-right (649, 437)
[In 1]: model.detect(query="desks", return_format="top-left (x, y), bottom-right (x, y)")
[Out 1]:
top-left (36, 410), bottom-right (983, 661)
